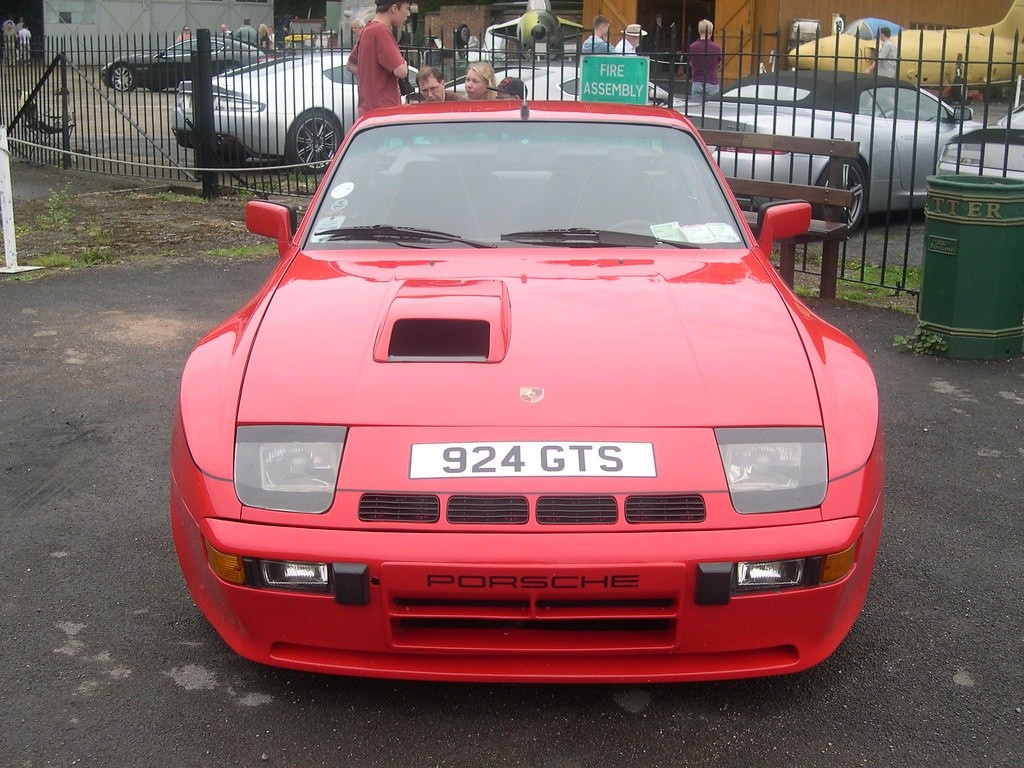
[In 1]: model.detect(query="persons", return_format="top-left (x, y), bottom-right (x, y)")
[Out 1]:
top-left (234, 18), bottom-right (257, 44)
top-left (486, 77), bottom-right (528, 100)
top-left (175, 26), bottom-right (193, 44)
top-left (582, 15), bottom-right (647, 56)
top-left (220, 24), bottom-right (231, 36)
top-left (277, 27), bottom-right (290, 40)
top-left (864, 27), bottom-right (897, 113)
top-left (347, 0), bottom-right (410, 118)
top-left (463, 62), bottom-right (497, 100)
top-left (259, 24), bottom-right (269, 47)
top-left (2, 15), bottom-right (31, 67)
top-left (416, 66), bottom-right (467, 102)
top-left (689, 19), bottom-right (721, 101)
top-left (293, 14), bottom-right (300, 19)
top-left (351, 18), bottom-right (366, 37)
top-left (267, 25), bottom-right (275, 48)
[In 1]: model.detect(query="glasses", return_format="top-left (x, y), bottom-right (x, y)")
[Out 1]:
top-left (420, 83), bottom-right (441, 94)
top-left (496, 96), bottom-right (514, 99)
top-left (184, 29), bottom-right (190, 31)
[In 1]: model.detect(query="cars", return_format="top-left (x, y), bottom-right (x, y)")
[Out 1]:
top-left (662, 69), bottom-right (1024, 240)
top-left (169, 46), bottom-right (686, 175)
top-left (101, 35), bottom-right (296, 92)
top-left (169, 101), bottom-right (886, 684)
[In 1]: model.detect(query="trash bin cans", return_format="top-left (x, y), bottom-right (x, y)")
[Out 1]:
top-left (915, 174), bottom-right (1024, 359)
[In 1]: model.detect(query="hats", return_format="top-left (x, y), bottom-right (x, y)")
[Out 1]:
top-left (487, 77), bottom-right (527, 100)
top-left (621, 24), bottom-right (647, 36)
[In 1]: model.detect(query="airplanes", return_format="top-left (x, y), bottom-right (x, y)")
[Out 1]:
top-left (486, 0), bottom-right (583, 60)
top-left (788, 0), bottom-right (1024, 86)
